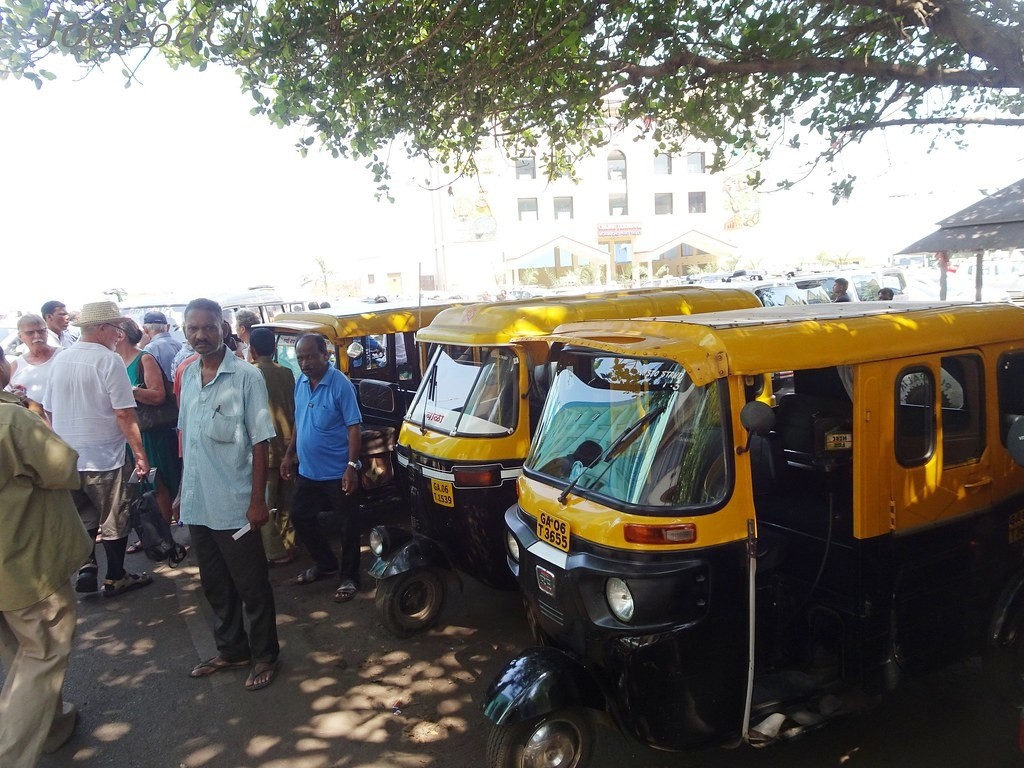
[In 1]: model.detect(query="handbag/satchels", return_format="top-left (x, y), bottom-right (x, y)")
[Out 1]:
top-left (131, 475), bottom-right (186, 563)
top-left (134, 353), bottom-right (179, 431)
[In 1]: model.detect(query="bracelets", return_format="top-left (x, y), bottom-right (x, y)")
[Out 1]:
top-left (348, 461), bottom-right (362, 470)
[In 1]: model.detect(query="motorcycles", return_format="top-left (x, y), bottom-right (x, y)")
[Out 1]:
top-left (478, 294), bottom-right (1024, 768)
top-left (366, 286), bottom-right (778, 638)
top-left (249, 296), bottom-right (489, 512)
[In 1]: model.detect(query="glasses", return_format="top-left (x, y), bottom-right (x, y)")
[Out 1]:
top-left (106, 322), bottom-right (125, 335)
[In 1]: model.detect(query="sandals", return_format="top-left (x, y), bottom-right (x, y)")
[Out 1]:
top-left (75, 564), bottom-right (98, 592)
top-left (297, 565), bottom-right (336, 584)
top-left (103, 569), bottom-right (152, 596)
top-left (334, 581), bottom-right (361, 601)
top-left (127, 540), bottom-right (142, 553)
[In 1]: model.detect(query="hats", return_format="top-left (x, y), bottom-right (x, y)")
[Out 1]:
top-left (71, 300), bottom-right (131, 327)
top-left (144, 310), bottom-right (168, 324)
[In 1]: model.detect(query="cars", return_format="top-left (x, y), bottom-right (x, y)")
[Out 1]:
top-left (0, 290), bottom-right (333, 362)
top-left (643, 254), bottom-right (1024, 393)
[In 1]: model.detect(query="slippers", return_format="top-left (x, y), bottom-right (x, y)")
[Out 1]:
top-left (189, 657), bottom-right (248, 678)
top-left (247, 655), bottom-right (281, 690)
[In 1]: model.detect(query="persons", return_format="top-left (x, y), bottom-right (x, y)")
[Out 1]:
top-left (877, 287), bottom-right (894, 300)
top-left (277, 332), bottom-right (363, 604)
top-left (0, 296), bottom-right (407, 692)
top-left (0, 391), bottom-right (96, 768)
top-left (832, 278), bottom-right (850, 302)
top-left (539, 440), bottom-right (602, 482)
